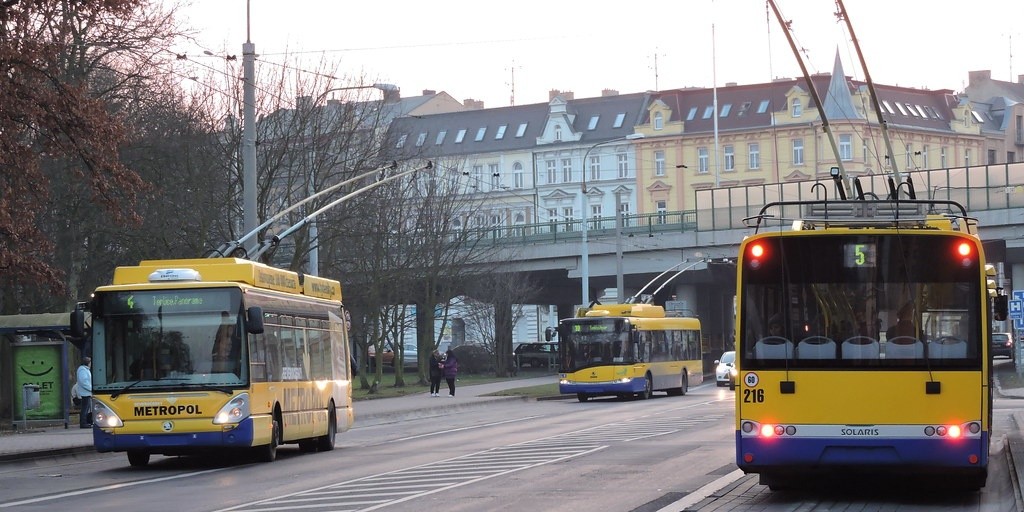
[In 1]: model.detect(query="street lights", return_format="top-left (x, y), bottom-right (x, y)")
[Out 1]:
top-left (580, 132), bottom-right (644, 308)
top-left (304, 83), bottom-right (401, 276)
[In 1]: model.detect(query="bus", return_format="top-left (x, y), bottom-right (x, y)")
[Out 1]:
top-left (67, 161), bottom-right (438, 463)
top-left (732, 0), bottom-right (1011, 507)
top-left (544, 258), bottom-right (711, 400)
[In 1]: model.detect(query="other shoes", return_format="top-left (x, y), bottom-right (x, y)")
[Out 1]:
top-left (431, 393), bottom-right (436, 397)
top-left (80, 424), bottom-right (92, 428)
top-left (448, 394), bottom-right (455, 397)
top-left (436, 393), bottom-right (440, 397)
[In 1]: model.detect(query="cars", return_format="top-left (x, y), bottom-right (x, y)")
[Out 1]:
top-left (364, 345), bottom-right (395, 368)
top-left (452, 344), bottom-right (496, 364)
top-left (713, 352), bottom-right (740, 387)
top-left (395, 343), bottom-right (418, 370)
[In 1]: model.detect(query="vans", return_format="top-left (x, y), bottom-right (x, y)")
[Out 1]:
top-left (513, 341), bottom-right (560, 368)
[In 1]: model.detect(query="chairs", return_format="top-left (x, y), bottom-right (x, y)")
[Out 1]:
top-left (754, 335), bottom-right (795, 359)
top-left (798, 337), bottom-right (837, 360)
top-left (886, 337), bottom-right (925, 360)
top-left (927, 336), bottom-right (969, 359)
top-left (843, 336), bottom-right (880, 359)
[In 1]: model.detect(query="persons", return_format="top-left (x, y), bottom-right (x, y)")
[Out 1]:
top-left (838, 310), bottom-right (882, 345)
top-left (388, 350), bottom-right (394, 359)
top-left (443, 350), bottom-right (457, 398)
top-left (71, 357), bottom-right (92, 429)
top-left (769, 311), bottom-right (784, 336)
top-left (140, 327), bottom-right (174, 370)
top-left (886, 301), bottom-right (916, 344)
top-left (220, 326), bottom-right (241, 357)
top-left (429, 350), bottom-right (444, 396)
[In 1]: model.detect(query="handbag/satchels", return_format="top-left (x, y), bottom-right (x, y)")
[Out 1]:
top-left (86, 412), bottom-right (93, 425)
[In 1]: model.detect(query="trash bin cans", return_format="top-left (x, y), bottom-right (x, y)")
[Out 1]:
top-left (26, 384), bottom-right (40, 410)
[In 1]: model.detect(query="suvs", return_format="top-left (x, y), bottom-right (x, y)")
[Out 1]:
top-left (992, 333), bottom-right (1013, 359)
